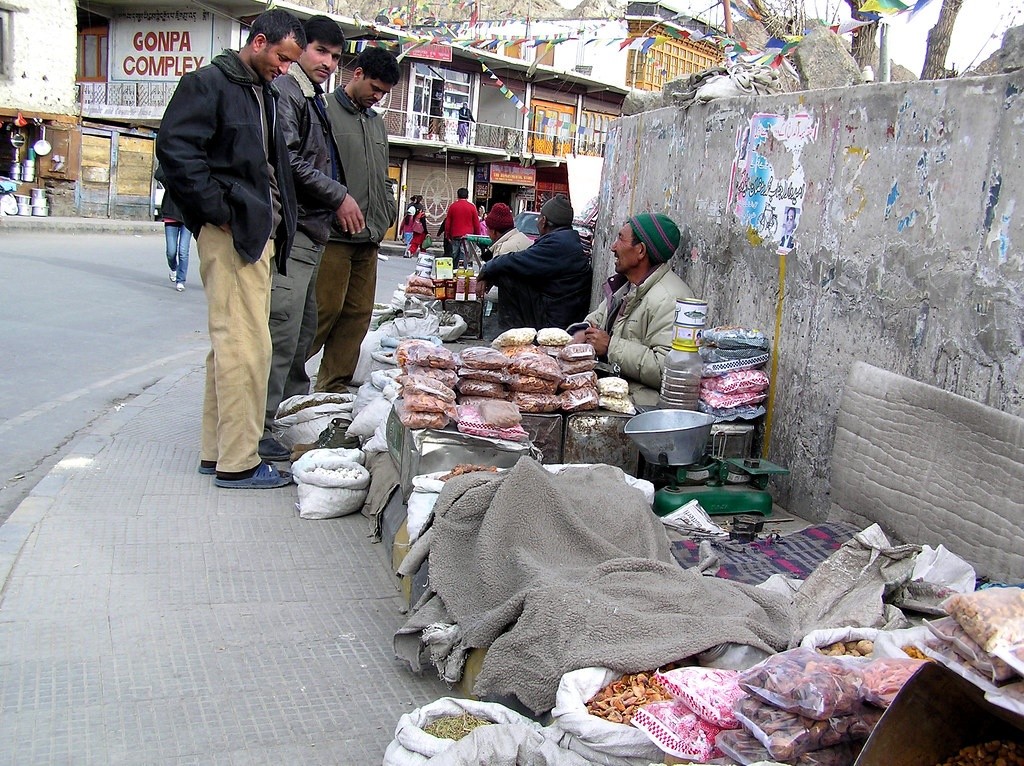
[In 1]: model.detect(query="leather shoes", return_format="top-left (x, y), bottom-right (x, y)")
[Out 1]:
top-left (258, 438), bottom-right (290, 460)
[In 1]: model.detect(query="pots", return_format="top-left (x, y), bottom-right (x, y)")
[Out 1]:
top-left (10, 123), bottom-right (49, 217)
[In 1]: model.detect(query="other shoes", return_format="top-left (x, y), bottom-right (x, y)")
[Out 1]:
top-left (176, 283), bottom-right (185, 292)
top-left (404, 254), bottom-right (413, 257)
top-left (169, 270), bottom-right (177, 281)
top-left (407, 251), bottom-right (411, 258)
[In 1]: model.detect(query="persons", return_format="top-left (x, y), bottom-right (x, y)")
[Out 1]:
top-left (400, 196), bottom-right (428, 258)
top-left (310, 46), bottom-right (401, 392)
top-left (457, 103), bottom-right (476, 143)
top-left (486, 203), bottom-right (533, 302)
top-left (156, 9), bottom-right (307, 488)
top-left (154, 163), bottom-right (192, 291)
top-left (476, 195), bottom-right (592, 344)
top-left (780, 207), bottom-right (797, 250)
top-left (259, 14), bottom-right (365, 460)
top-left (567, 214), bottom-right (695, 405)
top-left (436, 187), bottom-right (491, 269)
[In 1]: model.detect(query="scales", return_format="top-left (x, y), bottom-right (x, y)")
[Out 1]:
top-left (622, 410), bottom-right (791, 516)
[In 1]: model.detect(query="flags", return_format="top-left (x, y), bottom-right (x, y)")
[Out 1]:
top-left (346, 0), bottom-right (933, 134)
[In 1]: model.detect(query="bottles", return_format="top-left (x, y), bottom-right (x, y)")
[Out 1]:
top-left (458, 260), bottom-right (474, 300)
top-left (658, 340), bottom-right (703, 412)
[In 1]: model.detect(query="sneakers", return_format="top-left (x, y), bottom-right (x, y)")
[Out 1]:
top-left (290, 417), bottom-right (361, 464)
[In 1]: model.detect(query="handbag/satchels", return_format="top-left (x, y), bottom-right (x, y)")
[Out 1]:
top-left (422, 234), bottom-right (433, 249)
top-left (414, 221), bottom-right (423, 234)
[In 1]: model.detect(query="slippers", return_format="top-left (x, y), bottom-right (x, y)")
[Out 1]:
top-left (199, 457), bottom-right (277, 474)
top-left (215, 462), bottom-right (292, 488)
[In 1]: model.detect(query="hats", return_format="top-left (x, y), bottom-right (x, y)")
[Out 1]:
top-left (629, 213), bottom-right (681, 264)
top-left (541, 194), bottom-right (574, 225)
top-left (486, 203), bottom-right (515, 230)
